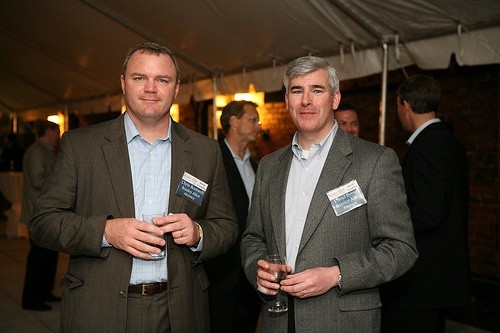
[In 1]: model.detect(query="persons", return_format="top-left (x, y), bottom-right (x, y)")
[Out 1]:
top-left (206, 100), bottom-right (265, 333)
top-left (21, 121), bottom-right (64, 310)
top-left (28, 41), bottom-right (239, 333)
top-left (332, 101), bottom-right (359, 139)
top-left (239, 56), bottom-right (418, 333)
top-left (396, 74), bottom-right (472, 333)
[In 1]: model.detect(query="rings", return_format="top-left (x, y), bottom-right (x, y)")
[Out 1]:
top-left (300, 291), bottom-right (304, 296)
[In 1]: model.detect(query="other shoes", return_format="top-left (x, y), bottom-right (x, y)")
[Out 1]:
top-left (21, 291), bottom-right (63, 311)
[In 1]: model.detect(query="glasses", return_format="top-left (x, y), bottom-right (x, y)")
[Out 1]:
top-left (247, 115), bottom-right (262, 128)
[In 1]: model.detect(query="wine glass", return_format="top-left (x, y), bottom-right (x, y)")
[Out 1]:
top-left (265, 254), bottom-right (288, 313)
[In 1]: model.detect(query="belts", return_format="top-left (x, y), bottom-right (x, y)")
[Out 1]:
top-left (127, 282), bottom-right (167, 297)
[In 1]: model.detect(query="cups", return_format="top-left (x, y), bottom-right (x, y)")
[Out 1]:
top-left (142, 212), bottom-right (166, 259)
